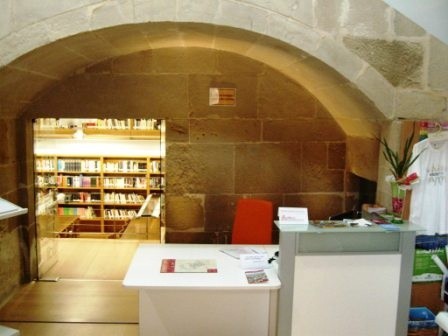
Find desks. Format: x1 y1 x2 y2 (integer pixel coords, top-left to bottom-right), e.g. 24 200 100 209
54 214 81 237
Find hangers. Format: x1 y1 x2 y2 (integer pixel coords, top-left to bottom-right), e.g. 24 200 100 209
430 121 448 144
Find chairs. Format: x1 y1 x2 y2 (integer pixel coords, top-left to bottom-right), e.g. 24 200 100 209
166 193 207 243
232 198 273 245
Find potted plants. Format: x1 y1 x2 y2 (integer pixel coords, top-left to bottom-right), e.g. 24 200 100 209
373 118 430 224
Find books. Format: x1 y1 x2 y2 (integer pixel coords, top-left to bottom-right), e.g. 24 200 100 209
150 177 161 188
34 159 54 171
36 174 101 188
103 176 146 188
103 159 147 172
150 160 160 173
103 192 145 203
104 209 137 219
58 159 101 172
57 191 101 203
58 208 96 220
159 258 218 273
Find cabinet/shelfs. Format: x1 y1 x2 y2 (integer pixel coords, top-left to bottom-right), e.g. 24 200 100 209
34 118 163 232
